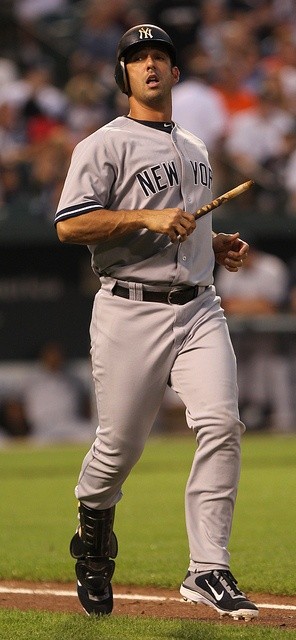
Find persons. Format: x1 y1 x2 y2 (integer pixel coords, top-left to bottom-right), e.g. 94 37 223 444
264 1 296 215
53 23 118 184
5 337 92 442
214 227 290 430
0 22 52 215
13 0 263 22
178 23 262 191
52 23 264 622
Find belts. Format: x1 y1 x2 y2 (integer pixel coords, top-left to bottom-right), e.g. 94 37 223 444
112 284 209 306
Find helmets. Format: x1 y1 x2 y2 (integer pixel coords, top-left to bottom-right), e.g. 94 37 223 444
113 24 175 96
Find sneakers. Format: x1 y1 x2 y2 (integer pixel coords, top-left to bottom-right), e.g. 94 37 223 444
180 569 259 622
75 559 114 617
